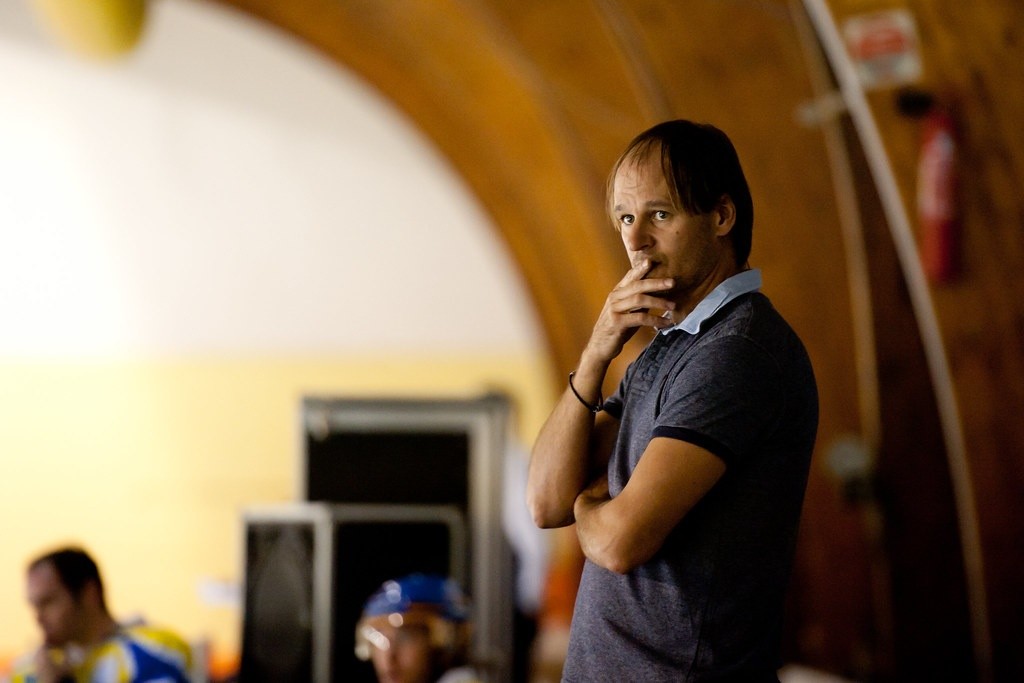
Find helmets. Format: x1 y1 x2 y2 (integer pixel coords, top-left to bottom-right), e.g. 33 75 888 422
354 574 475 658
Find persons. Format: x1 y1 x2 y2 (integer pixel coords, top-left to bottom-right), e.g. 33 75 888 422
12 547 213 683
355 575 487 683
525 120 819 683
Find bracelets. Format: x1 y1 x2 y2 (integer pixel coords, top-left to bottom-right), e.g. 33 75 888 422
570 371 605 414
